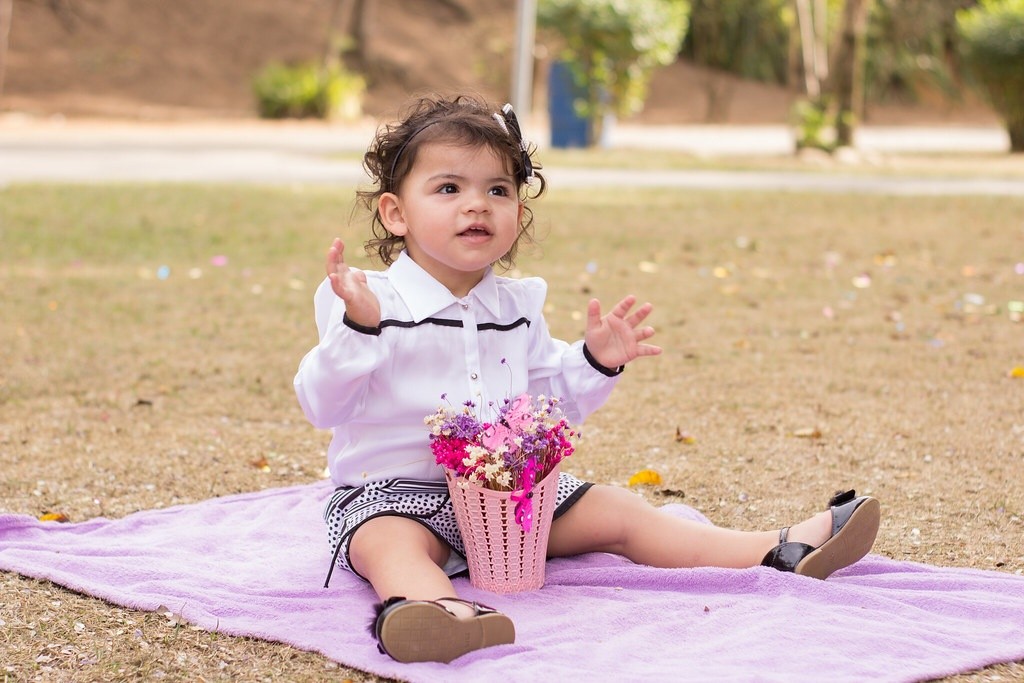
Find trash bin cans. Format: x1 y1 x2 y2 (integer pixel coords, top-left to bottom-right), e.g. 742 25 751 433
548 59 591 148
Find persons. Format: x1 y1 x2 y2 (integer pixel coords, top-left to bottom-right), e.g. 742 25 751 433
291 97 881 666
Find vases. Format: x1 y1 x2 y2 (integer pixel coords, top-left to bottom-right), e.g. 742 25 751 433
444 462 560 595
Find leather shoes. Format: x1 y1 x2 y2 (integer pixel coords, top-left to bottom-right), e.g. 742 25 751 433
364 596 515 663
761 488 880 580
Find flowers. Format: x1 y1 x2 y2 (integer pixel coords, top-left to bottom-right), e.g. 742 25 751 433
423 358 582 533
